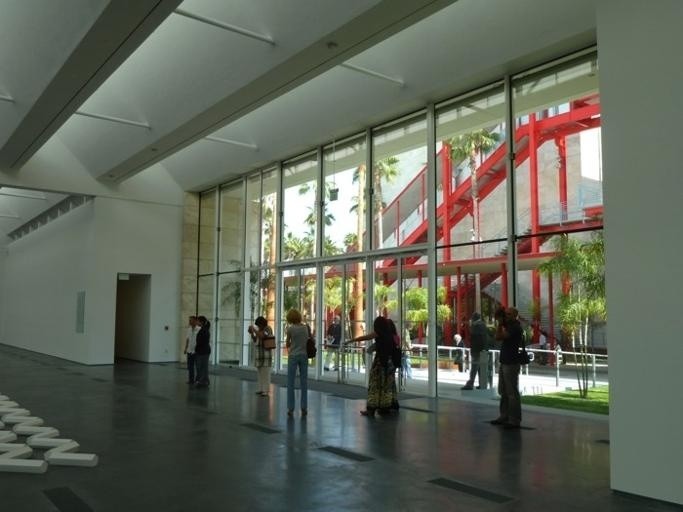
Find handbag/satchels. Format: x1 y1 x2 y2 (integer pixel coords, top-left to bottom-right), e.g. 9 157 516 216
519 350 530 365
263 335 276 349
393 347 402 368
307 339 317 358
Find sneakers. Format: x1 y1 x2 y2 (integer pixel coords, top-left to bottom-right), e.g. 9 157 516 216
360 409 398 416
505 423 521 428
287 410 293 418
302 408 307 416
187 380 208 386
460 386 472 389
491 420 504 424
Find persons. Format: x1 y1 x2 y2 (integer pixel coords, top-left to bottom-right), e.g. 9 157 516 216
184 316 201 384
453 312 534 390
342 316 413 419
553 338 563 366
194 316 211 386
539 330 547 365
286 309 310 415
248 316 273 397
491 307 524 430
324 315 342 371
